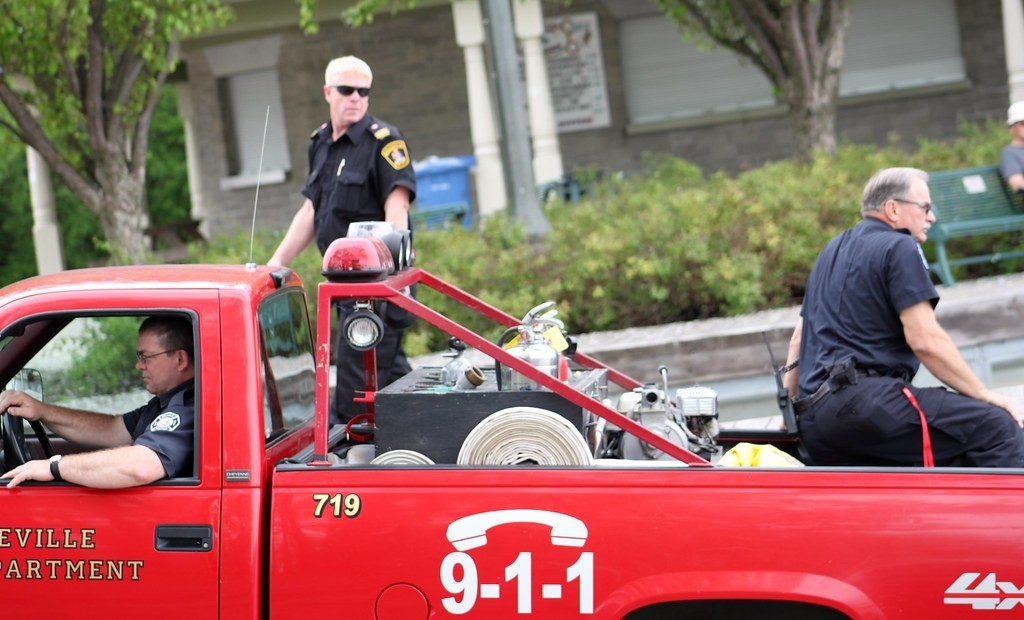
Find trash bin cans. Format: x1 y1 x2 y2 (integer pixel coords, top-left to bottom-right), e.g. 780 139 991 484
408 153 470 231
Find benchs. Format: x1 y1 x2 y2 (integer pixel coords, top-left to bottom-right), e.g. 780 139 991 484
918 164 1024 287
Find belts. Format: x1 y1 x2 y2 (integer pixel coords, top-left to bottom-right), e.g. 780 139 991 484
790 368 909 414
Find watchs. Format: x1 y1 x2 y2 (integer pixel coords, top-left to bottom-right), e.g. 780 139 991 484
49 454 61 477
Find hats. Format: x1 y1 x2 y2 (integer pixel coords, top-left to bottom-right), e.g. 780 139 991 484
1005 102 1024 125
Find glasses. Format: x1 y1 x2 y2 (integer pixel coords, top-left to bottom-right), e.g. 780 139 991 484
892 199 932 214
331 86 371 97
136 348 181 364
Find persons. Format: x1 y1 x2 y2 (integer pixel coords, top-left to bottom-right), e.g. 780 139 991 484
266 54 417 429
997 100 1024 193
0 312 195 489
781 167 1024 468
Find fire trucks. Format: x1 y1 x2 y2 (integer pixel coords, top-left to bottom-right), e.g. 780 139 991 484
0 222 1024 620
